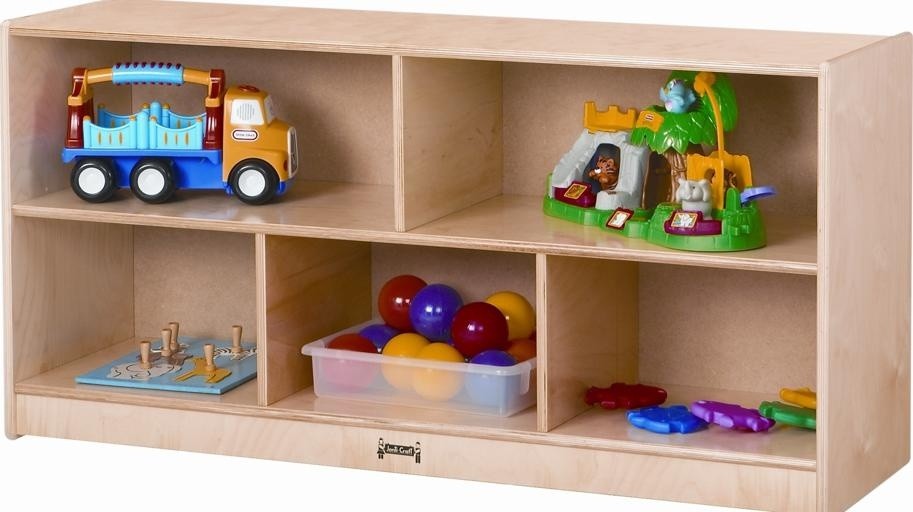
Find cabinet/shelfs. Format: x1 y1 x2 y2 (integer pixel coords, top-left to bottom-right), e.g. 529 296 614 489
0 0 913 512
